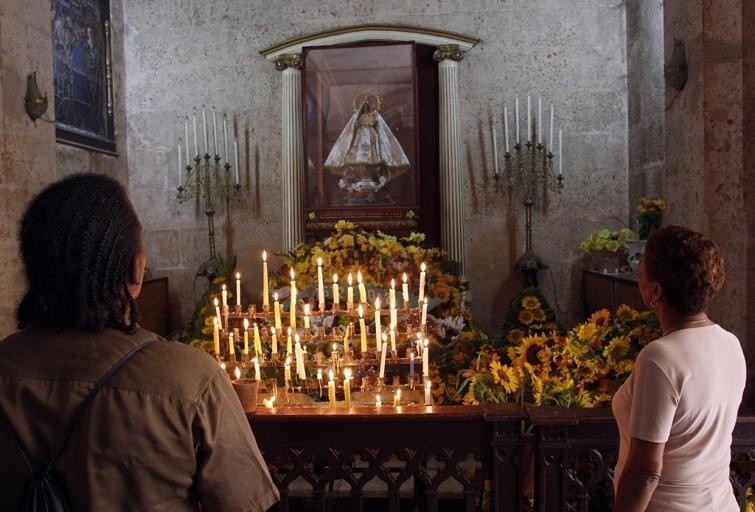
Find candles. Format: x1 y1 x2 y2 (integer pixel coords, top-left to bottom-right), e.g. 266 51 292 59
211 250 433 409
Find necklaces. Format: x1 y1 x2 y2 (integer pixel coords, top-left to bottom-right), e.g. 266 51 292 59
661 318 709 336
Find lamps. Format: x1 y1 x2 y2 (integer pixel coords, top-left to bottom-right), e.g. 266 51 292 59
175 104 241 261
492 92 564 288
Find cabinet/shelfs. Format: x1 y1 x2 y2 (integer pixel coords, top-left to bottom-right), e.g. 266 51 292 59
573 267 648 326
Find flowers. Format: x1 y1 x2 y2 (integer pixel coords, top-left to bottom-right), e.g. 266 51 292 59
636 198 665 239
580 228 637 257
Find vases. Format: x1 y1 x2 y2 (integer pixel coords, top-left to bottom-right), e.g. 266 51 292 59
590 246 631 273
625 240 648 273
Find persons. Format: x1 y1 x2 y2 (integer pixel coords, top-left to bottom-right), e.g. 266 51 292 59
0 175 280 511
612 225 747 512
